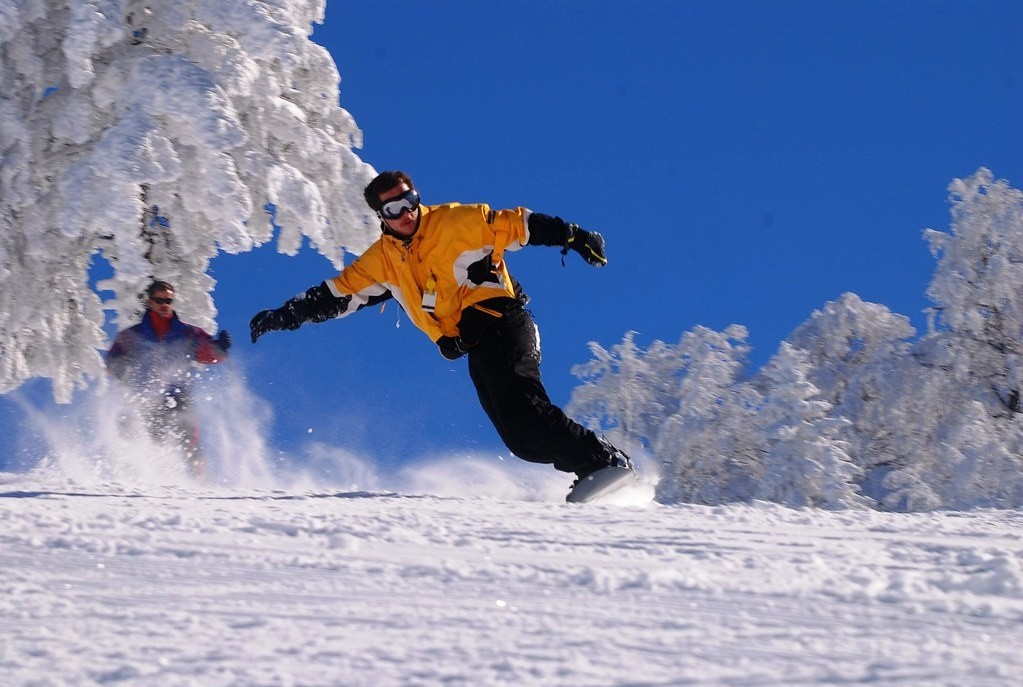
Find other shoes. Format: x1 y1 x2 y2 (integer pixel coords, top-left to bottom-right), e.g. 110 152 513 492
570 439 629 485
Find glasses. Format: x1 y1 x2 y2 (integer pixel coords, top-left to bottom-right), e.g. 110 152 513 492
149 297 173 304
380 189 420 220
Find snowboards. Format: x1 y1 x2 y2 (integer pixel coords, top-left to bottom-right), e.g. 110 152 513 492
566 466 635 503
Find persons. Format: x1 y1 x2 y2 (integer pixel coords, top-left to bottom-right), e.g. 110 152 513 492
250 170 631 489
103 281 232 475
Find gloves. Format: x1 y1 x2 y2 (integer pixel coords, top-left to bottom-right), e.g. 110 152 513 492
250 301 301 343
218 329 232 353
550 216 608 268
